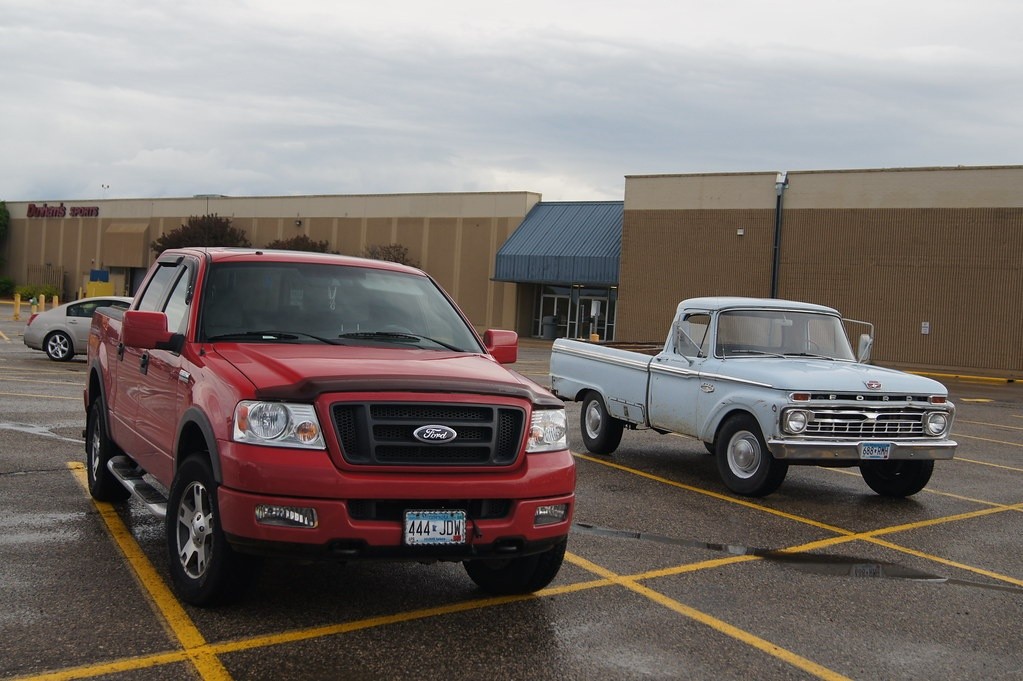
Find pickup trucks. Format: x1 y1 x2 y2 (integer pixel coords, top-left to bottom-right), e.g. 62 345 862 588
83 247 576 609
550 296 958 497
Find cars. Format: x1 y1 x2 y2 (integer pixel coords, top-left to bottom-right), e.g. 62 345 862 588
24 297 135 362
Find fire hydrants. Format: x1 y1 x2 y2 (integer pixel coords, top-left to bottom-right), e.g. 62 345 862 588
29 297 40 314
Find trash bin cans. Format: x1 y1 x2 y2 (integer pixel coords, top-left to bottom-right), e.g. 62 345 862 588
542 315 558 340
